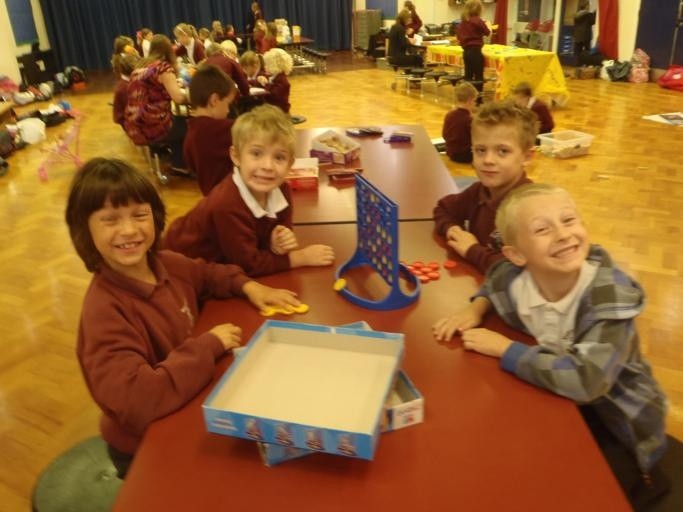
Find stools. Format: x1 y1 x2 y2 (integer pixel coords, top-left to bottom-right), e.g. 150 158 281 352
140 140 175 186
293 44 333 74
32 433 125 512
384 63 498 109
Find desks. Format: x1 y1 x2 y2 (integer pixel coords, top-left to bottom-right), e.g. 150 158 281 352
16 49 56 86
108 218 635 512
424 43 572 112
232 31 316 71
278 123 462 225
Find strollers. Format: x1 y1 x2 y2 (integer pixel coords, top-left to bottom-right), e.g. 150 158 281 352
3 109 89 183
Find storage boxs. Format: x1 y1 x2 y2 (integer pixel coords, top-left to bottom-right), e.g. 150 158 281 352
535 129 595 160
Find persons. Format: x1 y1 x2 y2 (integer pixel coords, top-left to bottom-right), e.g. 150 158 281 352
65 0 668 507
